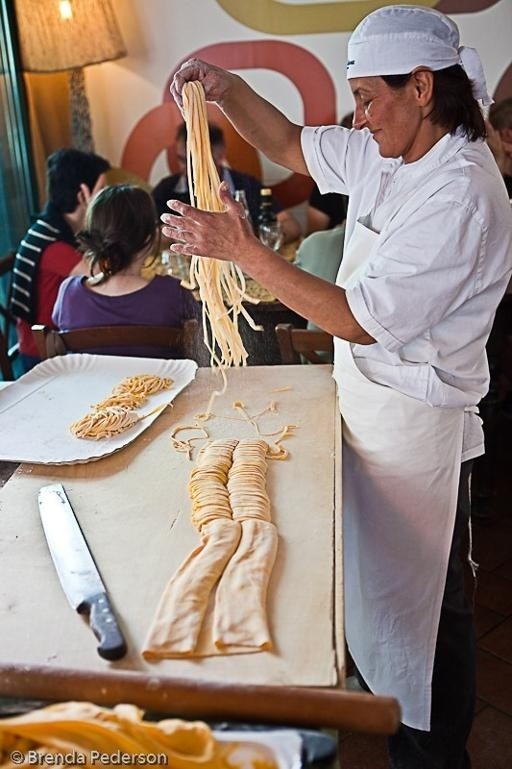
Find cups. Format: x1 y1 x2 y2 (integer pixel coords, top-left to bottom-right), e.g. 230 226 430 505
258 225 283 253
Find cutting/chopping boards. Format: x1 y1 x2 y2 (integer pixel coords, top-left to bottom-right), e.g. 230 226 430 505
0 364 345 691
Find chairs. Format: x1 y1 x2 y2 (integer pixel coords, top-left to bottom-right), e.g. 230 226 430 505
38 314 336 368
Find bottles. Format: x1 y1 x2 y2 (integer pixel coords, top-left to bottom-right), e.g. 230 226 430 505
233 189 252 226
258 189 277 249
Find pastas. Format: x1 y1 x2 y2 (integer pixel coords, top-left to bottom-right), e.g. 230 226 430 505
71 373 175 440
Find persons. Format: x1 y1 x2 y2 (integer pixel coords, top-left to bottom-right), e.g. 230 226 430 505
160 3 512 769
9 100 512 373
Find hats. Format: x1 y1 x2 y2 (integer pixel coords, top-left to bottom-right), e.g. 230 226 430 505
344 4 461 83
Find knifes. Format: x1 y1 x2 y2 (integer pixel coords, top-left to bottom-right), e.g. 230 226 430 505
36 481 128 660
